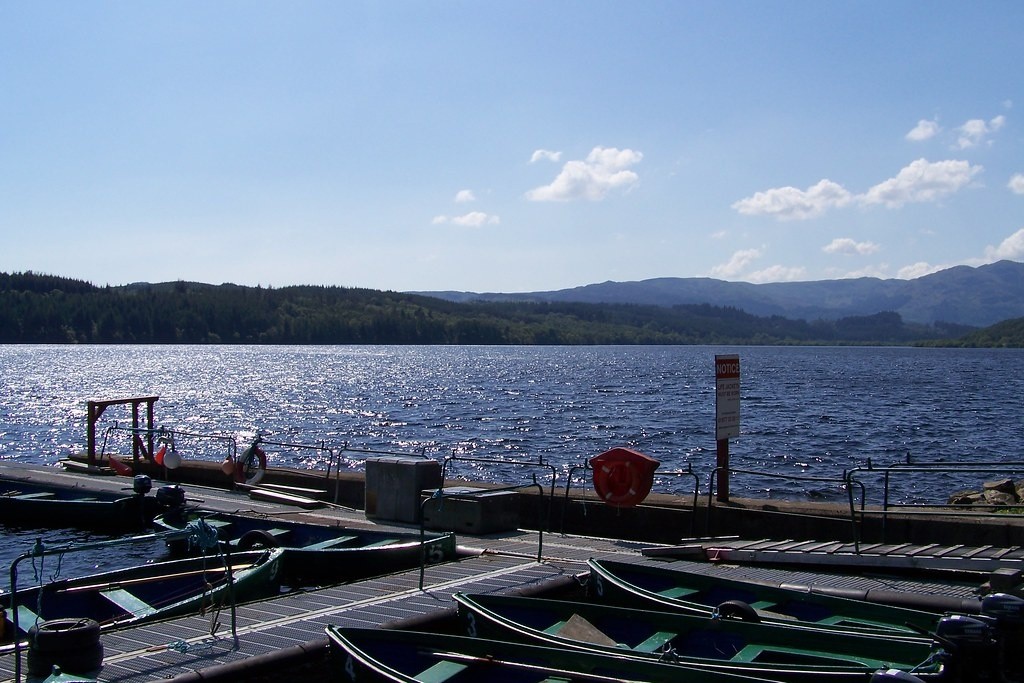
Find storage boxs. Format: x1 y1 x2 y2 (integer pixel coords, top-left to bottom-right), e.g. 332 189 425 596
365 456 442 522
418 486 522 534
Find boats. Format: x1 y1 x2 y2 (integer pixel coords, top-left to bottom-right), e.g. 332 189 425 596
0 477 456 647
325 557 1024 682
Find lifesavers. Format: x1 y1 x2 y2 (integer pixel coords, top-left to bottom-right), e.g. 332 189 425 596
236 530 282 582
27 643 104 674
236 448 266 491
26 618 101 650
708 600 760 659
600 461 639 502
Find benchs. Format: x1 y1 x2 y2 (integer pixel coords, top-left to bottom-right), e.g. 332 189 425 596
301 536 359 549
747 601 778 610
228 529 292 545
4 604 44 634
209 520 233 527
229 568 249 579
655 586 699 599
543 620 569 635
729 644 769 662
633 631 679 652
362 538 402 549
74 498 98 501
815 614 846 624
539 676 572 683
99 587 158 618
414 658 469 683
14 492 56 497
